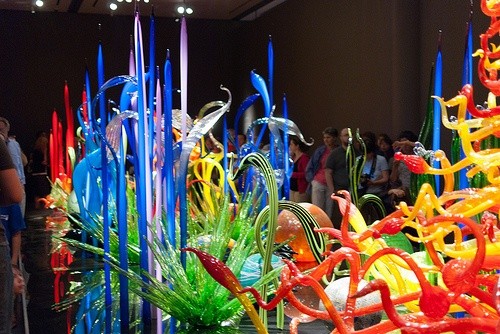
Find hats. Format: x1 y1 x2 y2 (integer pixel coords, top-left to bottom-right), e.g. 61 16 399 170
359 136 374 154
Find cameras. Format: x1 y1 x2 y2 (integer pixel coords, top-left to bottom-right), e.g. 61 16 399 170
359 172 368 186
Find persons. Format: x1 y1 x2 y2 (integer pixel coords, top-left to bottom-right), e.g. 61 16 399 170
229 127 418 227
0 117 27 334
